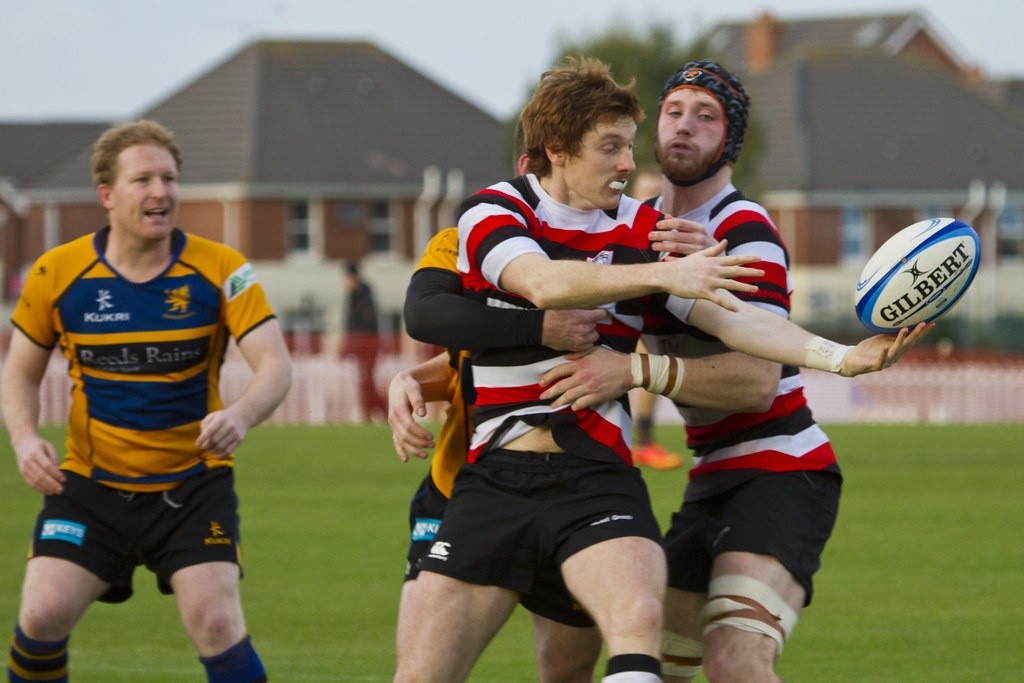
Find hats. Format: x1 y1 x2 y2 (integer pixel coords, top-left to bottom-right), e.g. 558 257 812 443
654 59 751 186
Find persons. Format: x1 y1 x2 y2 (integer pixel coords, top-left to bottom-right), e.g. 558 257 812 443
331 262 388 425
0 120 292 682
387 61 843 683
393 66 936 683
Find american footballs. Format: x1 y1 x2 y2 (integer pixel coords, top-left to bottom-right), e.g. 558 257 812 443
854 216 981 334
